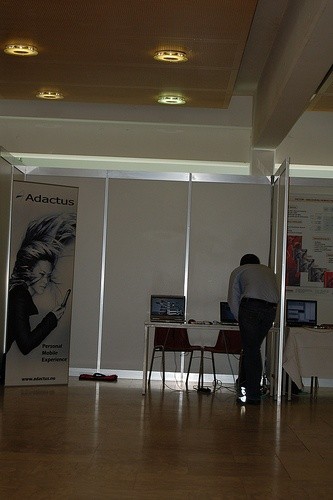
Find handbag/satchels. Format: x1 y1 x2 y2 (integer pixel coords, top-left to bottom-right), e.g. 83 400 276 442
78 373 118 382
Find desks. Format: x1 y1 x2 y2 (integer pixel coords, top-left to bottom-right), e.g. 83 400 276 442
142 321 280 401
283 327 333 401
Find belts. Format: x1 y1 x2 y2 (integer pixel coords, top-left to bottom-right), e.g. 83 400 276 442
242 298 277 306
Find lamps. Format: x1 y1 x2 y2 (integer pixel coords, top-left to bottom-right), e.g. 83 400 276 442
154 46 189 63
2 38 40 57
154 91 191 105
33 88 64 100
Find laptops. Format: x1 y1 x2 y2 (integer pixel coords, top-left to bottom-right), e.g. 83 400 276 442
286 299 319 328
220 302 239 326
150 295 185 325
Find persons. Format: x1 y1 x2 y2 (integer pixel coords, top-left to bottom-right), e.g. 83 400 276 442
0 214 76 355
227 254 279 402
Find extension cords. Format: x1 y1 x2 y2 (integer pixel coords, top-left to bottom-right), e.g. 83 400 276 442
198 388 211 392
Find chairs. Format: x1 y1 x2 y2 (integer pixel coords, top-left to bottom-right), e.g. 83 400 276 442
149 327 203 392
198 330 241 391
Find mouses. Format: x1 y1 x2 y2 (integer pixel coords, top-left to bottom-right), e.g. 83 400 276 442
188 319 196 324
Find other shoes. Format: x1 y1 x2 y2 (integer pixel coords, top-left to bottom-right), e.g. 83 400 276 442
236 397 261 406
236 379 248 389
